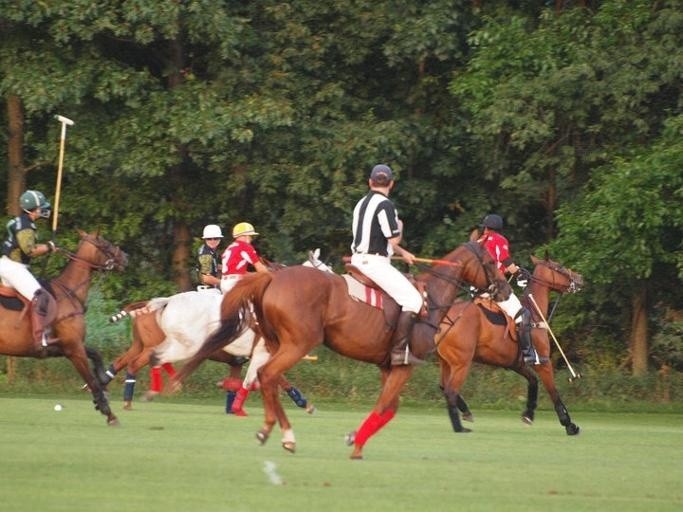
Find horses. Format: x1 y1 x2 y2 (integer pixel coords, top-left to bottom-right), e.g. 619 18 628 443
0 225 130 426
81 258 316 414
435 249 584 436
162 225 513 459
128 248 337 417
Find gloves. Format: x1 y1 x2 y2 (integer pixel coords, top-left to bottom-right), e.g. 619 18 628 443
48 240 57 252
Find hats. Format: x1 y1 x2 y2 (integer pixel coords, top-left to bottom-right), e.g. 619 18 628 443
370 164 393 181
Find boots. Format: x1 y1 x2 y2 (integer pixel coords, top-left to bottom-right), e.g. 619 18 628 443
515 321 548 365
391 311 427 365
31 300 60 352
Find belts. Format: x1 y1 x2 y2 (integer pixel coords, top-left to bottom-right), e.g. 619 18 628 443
201 287 214 289
223 276 241 279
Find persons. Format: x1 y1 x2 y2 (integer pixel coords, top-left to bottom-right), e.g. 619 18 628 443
466 213 548 370
221 218 277 334
195 224 225 296
346 160 427 371
2 185 66 349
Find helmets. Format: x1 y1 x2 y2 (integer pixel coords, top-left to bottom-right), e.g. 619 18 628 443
202 224 224 238
483 214 503 232
20 189 50 210
233 222 260 238
